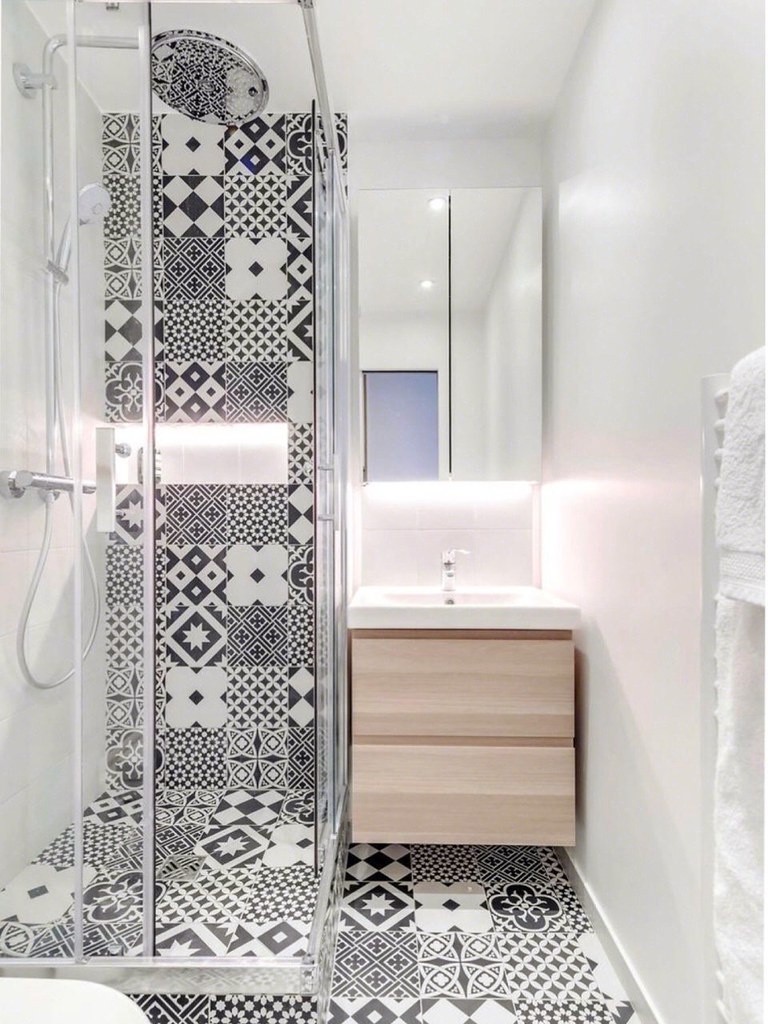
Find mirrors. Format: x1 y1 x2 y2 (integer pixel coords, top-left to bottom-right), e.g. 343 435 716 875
356 189 542 480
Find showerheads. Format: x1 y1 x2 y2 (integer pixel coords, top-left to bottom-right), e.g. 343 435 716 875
150 28 270 126
52 182 114 284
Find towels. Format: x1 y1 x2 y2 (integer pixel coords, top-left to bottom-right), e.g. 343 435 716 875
717 344 765 608
712 342 767 1024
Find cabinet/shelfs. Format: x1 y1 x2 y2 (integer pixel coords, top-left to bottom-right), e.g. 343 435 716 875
347 609 577 847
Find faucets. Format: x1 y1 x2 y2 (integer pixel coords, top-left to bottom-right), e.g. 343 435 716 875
14 468 96 501
436 546 469 599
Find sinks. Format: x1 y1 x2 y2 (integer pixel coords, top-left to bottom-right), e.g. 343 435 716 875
345 581 583 632
